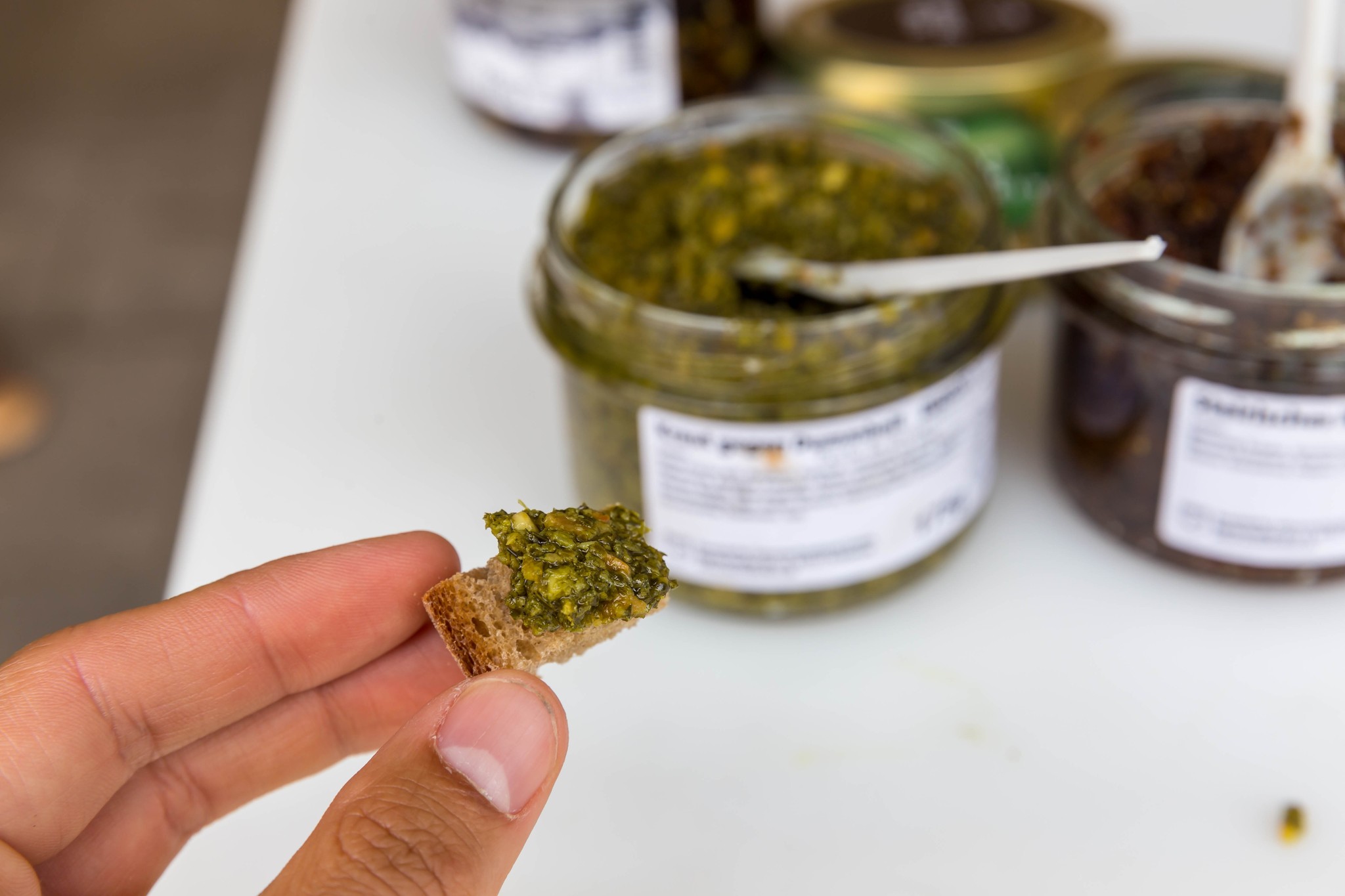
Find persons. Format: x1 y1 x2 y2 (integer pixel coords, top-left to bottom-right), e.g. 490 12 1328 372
2 533 570 896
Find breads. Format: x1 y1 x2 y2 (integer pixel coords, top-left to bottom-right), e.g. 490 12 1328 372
422 504 678 678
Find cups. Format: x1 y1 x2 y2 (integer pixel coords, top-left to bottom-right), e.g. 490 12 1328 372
437 0 677 142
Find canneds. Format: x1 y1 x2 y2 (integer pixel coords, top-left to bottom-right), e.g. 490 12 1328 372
788 0 1114 288
1044 59 1345 585
447 0 766 141
522 90 1012 616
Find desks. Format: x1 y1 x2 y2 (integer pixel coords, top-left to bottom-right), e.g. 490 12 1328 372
145 1 1345 896
